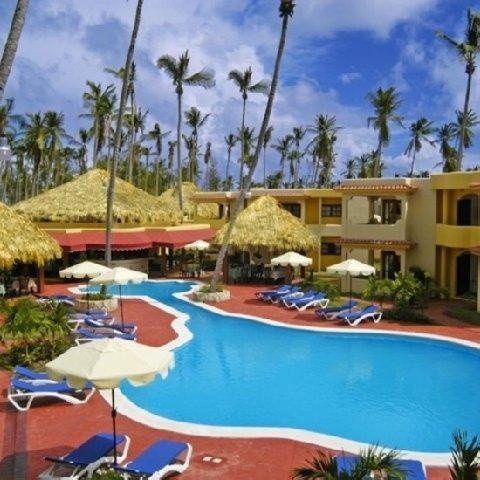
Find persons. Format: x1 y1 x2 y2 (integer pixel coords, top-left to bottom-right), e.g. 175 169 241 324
184 250 194 278
11 279 21 297
24 272 38 293
0 272 6 296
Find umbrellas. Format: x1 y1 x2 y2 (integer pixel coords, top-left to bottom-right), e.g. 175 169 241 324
58 261 111 311
271 251 313 293
45 336 176 467
325 258 377 313
89 266 150 334
185 240 211 252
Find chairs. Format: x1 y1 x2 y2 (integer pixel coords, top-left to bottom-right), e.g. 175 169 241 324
331 455 427 480
37 430 193 480
254 286 382 327
3 277 138 411
